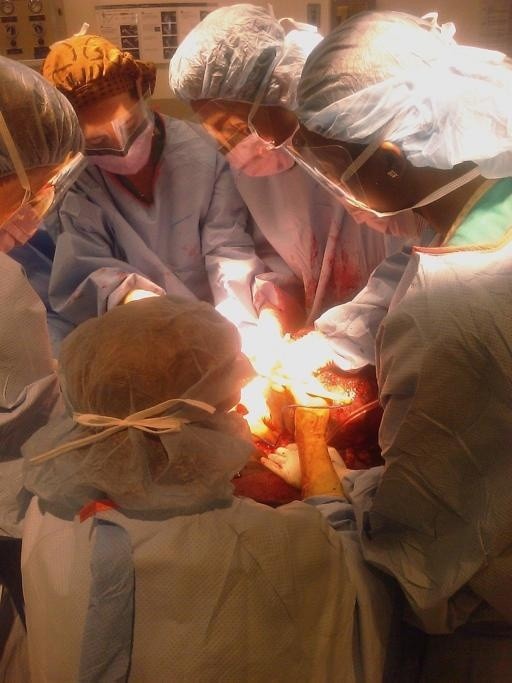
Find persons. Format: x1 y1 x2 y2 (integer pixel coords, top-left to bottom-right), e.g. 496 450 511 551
253 8 511 643
167 3 442 383
1 54 92 541
5 19 272 359
0 293 396 682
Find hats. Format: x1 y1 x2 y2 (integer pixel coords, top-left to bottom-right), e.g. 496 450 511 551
42 34 156 115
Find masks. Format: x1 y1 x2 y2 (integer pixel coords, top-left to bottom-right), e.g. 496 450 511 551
336 144 428 240
225 101 300 178
85 77 155 176
0 170 44 255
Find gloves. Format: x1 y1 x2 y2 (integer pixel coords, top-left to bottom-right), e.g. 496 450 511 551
259 442 349 491
